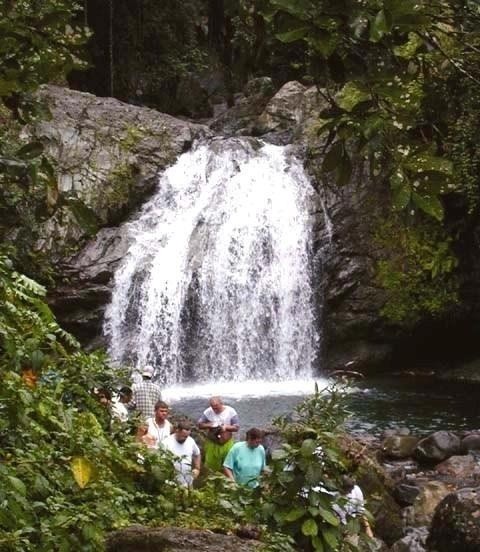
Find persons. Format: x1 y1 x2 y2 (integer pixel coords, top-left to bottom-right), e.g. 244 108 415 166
197 397 240 444
161 422 201 488
223 427 266 487
132 365 162 419
94 369 133 435
283 437 373 545
137 401 172 451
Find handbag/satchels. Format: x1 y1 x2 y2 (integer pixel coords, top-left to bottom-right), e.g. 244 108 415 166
216 429 233 445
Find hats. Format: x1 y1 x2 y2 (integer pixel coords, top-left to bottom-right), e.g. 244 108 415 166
142 365 154 378
